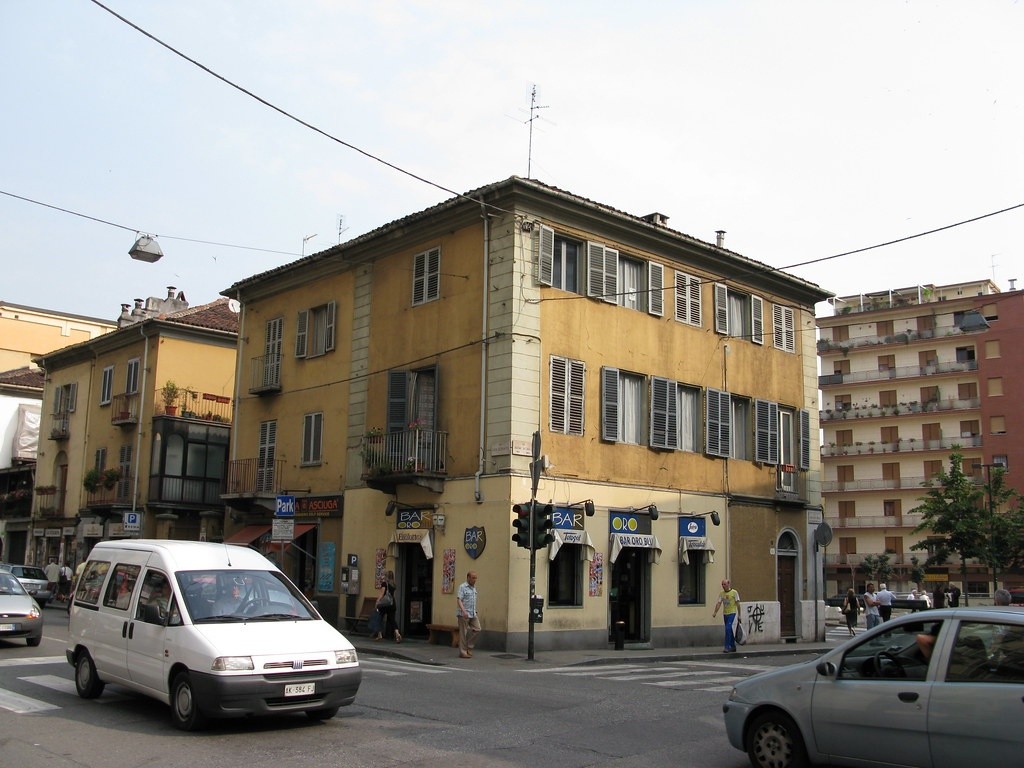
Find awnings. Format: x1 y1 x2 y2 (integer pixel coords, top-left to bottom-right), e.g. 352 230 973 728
269 524 316 559
221 524 272 556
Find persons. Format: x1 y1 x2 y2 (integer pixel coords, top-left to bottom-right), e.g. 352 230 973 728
44 558 63 602
990 589 1012 650
946 583 960 608
877 583 897 622
933 584 945 609
919 590 932 610
373 571 402 643
712 579 742 653
59 562 73 600
916 623 944 660
907 590 917 613
456 572 481 658
863 583 881 631
209 580 250 616
75 555 91 580
843 588 860 637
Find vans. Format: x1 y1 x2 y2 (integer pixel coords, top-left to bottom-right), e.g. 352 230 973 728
0 568 44 647
65 538 362 732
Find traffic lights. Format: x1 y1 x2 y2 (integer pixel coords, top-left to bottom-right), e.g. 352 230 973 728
512 502 530 547
533 503 554 549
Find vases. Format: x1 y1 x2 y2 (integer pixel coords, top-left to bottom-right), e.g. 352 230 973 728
414 430 424 438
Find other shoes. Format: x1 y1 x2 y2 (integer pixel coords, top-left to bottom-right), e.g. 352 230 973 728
376 636 383 640
467 650 472 656
460 652 471 658
396 634 402 643
49 596 54 603
852 629 856 636
723 649 729 653
61 596 66 603
728 648 736 652
849 634 852 637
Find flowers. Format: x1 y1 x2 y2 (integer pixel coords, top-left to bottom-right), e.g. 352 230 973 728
408 419 427 432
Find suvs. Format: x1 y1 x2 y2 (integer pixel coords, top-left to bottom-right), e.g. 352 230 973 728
0 561 53 609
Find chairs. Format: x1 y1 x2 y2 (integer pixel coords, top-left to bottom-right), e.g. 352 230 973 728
961 636 995 682
996 631 1024 683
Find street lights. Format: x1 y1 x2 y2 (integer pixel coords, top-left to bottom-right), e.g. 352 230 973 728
971 461 1006 606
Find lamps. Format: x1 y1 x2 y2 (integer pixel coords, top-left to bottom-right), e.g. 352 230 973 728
959 310 991 332
128 235 164 263
567 499 595 516
634 502 659 520
696 511 720 526
385 500 416 516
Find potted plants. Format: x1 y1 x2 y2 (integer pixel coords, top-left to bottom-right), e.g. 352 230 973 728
34 485 57 495
405 457 424 471
180 385 232 423
120 388 130 420
162 380 179 416
358 426 392 472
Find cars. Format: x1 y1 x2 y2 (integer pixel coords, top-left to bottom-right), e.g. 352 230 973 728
723 606 1024 768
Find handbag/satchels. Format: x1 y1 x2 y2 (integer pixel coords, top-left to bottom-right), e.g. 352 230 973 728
842 603 851 615
376 593 393 611
59 575 67 585
734 621 746 645
